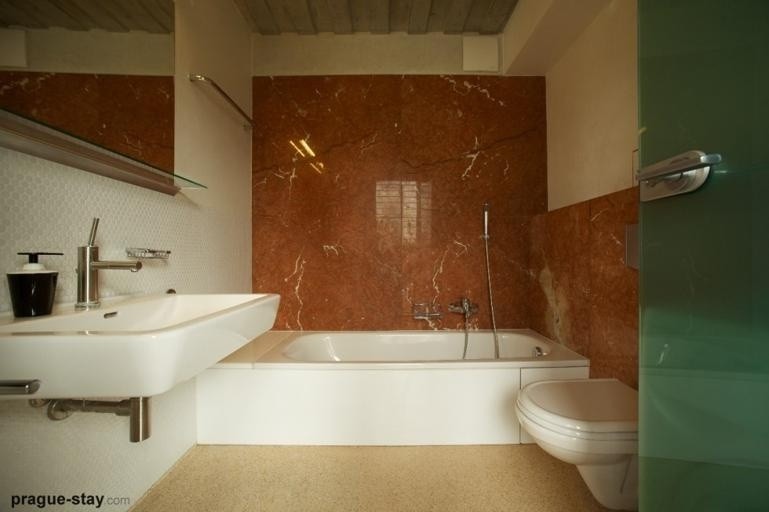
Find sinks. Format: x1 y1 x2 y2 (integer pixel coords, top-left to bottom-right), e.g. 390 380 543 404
0 291 280 401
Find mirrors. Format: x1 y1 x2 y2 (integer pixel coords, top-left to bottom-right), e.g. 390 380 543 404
1 0 207 197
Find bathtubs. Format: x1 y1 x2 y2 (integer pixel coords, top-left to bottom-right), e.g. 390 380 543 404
196 329 591 446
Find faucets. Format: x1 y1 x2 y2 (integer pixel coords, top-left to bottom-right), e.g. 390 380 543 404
76 218 141 308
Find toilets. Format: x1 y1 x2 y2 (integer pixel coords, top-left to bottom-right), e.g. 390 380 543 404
516 377 638 511
